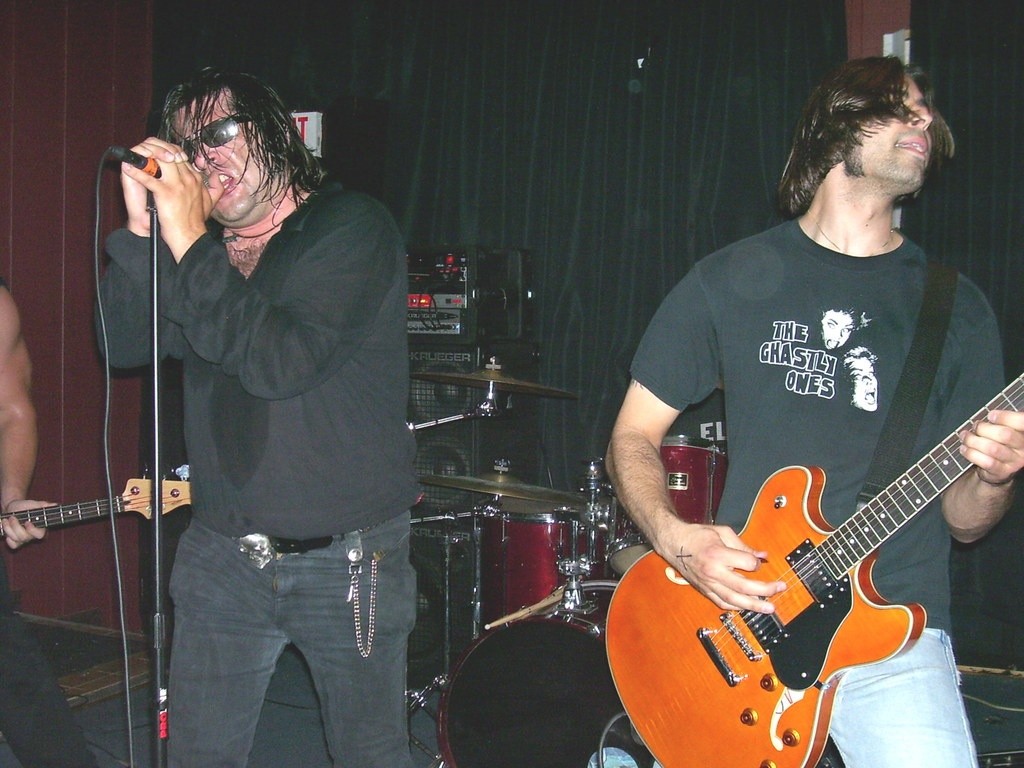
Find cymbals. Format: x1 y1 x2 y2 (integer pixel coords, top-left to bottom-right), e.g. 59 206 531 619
412 360 567 398
415 464 585 507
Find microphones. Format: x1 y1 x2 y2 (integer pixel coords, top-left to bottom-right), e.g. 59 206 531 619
108 146 210 188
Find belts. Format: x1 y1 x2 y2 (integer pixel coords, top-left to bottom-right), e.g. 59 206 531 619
238 525 372 570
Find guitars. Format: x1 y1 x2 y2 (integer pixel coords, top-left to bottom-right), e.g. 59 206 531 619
605 370 1024 766
0 465 190 536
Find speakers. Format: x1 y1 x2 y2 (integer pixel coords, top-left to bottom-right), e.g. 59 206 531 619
407 339 542 673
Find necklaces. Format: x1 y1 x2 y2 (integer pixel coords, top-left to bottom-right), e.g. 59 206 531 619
221 222 286 243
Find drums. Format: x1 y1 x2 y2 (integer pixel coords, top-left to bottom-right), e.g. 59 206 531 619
609 435 727 574
955 662 1024 768
503 510 608 637
437 603 624 767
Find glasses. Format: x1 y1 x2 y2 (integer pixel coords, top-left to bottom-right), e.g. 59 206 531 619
178 115 252 164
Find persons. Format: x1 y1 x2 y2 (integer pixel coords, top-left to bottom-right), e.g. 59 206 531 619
601 55 1024 768
91 61 425 768
0 281 102 768
